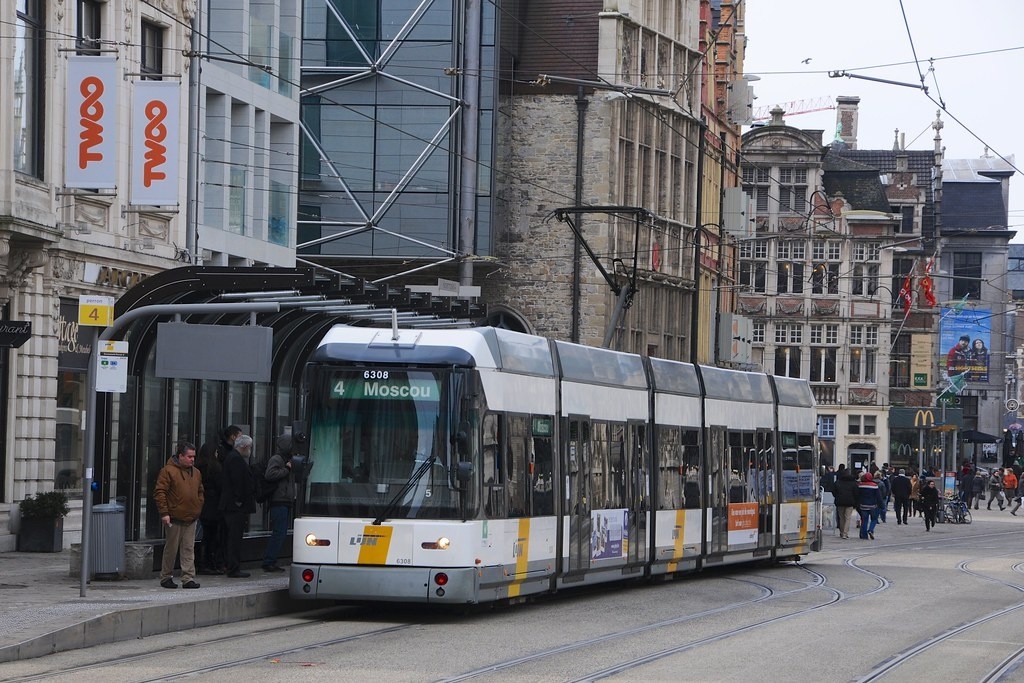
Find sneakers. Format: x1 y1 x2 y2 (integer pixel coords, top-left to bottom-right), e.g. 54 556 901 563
183 581 200 588
161 579 178 588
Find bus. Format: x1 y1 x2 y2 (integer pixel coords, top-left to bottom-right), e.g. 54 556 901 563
288 206 823 607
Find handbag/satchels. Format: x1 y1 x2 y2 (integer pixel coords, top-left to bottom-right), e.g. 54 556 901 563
999 490 1006 501
980 494 985 500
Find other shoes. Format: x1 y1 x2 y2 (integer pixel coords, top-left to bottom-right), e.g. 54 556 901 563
228 571 250 578
869 531 875 540
974 506 979 510
840 532 849 539
1011 510 1016 516
266 567 284 573
198 567 224 575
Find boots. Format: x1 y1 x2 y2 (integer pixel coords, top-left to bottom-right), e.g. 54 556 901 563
987 503 991 510
998 503 1005 510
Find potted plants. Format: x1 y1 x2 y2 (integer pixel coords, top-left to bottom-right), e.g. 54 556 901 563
17 491 71 553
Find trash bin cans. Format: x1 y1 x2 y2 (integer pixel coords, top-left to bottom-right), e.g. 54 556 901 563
90 504 125 582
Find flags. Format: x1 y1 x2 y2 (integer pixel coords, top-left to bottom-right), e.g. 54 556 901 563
920 260 935 308
899 273 914 318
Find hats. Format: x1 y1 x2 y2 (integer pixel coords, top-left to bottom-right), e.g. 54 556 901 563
959 334 970 343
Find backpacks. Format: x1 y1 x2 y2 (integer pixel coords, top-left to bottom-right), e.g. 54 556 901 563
250 451 287 504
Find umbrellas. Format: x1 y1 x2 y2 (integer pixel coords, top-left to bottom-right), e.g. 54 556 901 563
955 429 1002 463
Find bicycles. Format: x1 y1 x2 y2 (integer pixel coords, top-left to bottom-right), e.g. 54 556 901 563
946 491 972 524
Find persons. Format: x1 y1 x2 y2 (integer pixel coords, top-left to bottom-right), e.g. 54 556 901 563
154 441 204 589
200 424 293 578
819 448 1024 541
946 336 989 380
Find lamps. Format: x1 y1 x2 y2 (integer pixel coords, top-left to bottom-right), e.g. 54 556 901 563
185 249 212 261
63 222 91 235
128 236 155 249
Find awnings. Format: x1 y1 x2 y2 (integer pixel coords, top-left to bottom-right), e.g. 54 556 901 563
887 405 964 429
